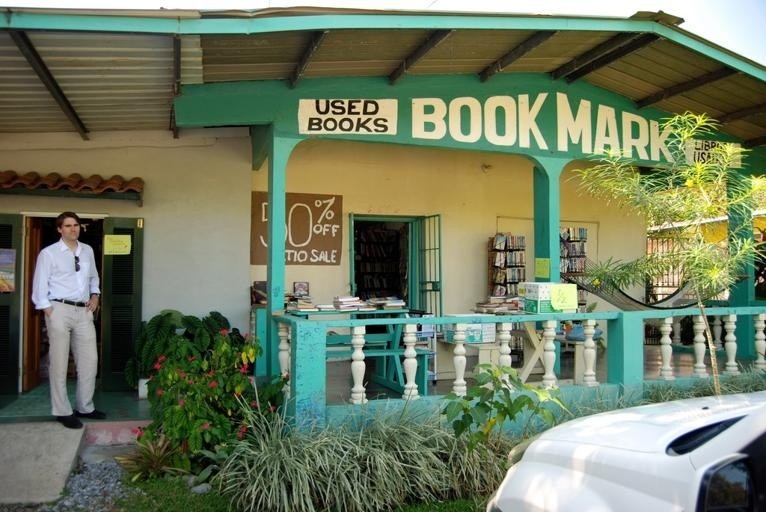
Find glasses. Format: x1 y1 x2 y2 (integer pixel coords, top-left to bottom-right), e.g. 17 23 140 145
75 256 80 271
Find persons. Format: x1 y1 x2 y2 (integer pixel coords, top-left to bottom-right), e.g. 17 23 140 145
31 210 107 429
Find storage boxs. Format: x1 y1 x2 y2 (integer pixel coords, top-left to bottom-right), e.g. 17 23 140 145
443 313 496 344
518 282 578 315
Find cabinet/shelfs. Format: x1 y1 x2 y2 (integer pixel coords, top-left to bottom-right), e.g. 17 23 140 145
560 238 587 353
487 236 527 367
354 221 408 319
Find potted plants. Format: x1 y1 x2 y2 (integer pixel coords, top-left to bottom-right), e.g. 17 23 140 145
124 310 232 399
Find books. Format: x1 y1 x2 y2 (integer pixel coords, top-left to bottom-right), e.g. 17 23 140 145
251 226 406 317
487 232 526 351
560 227 588 312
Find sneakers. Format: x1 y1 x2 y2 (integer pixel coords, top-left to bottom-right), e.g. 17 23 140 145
56 415 82 428
75 409 106 419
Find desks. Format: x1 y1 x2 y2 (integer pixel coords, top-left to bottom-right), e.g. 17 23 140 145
498 310 547 385
289 309 409 394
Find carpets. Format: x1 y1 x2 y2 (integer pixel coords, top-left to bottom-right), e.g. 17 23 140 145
0 380 100 418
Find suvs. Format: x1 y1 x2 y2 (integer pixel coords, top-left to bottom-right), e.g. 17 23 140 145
484 390 766 512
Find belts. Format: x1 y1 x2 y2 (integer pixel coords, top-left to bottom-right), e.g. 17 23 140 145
54 299 85 307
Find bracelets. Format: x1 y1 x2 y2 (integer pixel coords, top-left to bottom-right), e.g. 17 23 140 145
90 293 100 299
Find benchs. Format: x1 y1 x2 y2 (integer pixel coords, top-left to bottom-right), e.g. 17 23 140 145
437 339 501 374
496 329 597 385
326 349 429 396
326 334 392 385
